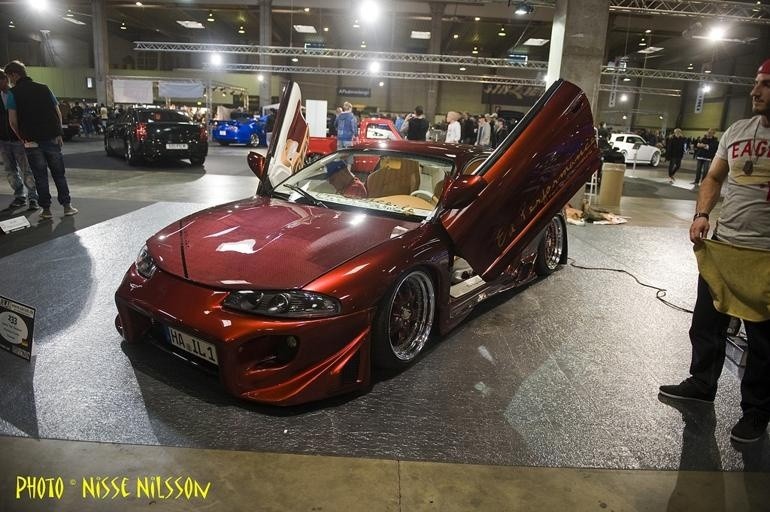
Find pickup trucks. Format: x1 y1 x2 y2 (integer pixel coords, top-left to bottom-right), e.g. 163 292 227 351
304 118 404 174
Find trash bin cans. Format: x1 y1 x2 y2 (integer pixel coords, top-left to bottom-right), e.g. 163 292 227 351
600 163 625 213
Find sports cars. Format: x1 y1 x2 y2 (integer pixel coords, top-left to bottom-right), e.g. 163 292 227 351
112 76 604 407
208 116 268 147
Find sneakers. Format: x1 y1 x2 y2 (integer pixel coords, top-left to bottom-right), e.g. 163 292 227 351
8 198 27 208
63 205 78 217
28 200 39 211
39 209 53 219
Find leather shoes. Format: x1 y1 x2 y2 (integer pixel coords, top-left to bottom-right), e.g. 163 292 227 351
660 374 719 404
731 415 765 444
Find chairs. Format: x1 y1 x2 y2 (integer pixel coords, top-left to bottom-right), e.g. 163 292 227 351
385 159 419 195
463 160 486 177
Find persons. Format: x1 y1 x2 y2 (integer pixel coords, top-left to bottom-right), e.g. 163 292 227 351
324 101 720 199
0 59 78 219
660 62 769 444
62 100 276 148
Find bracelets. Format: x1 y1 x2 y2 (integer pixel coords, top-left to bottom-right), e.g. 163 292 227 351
694 212 711 222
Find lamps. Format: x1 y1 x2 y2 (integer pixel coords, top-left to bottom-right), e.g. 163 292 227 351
515 4 532 16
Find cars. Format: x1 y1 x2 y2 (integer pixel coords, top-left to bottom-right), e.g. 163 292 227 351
611 132 661 167
104 104 209 167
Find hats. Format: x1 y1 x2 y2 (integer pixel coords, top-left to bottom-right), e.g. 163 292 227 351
758 59 770 75
